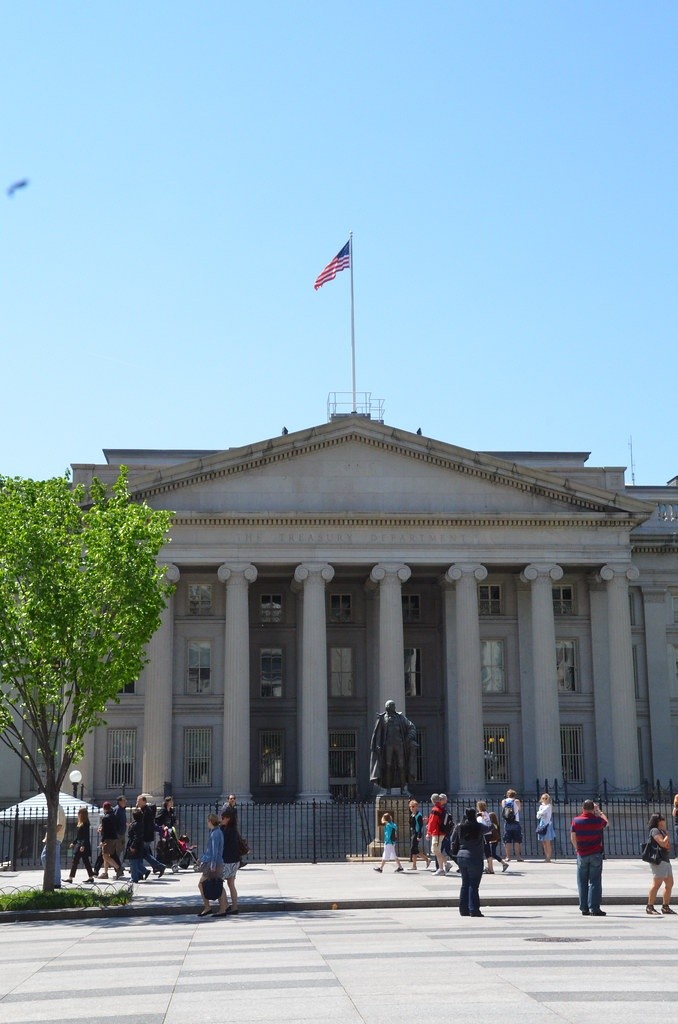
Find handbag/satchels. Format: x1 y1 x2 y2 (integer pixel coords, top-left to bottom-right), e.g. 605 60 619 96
239 836 251 856
535 824 547 836
201 870 224 900
640 828 663 865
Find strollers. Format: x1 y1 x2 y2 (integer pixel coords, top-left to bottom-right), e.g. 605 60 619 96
160 826 202 874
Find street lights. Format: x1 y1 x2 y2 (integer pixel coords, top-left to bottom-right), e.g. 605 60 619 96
69 770 83 797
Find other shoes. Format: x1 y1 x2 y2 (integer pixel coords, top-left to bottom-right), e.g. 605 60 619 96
97 873 108 879
461 912 475 917
145 869 151 880
137 875 144 880
63 879 72 883
582 910 590 915
470 911 484 917
661 906 677 914
198 909 212 916
646 904 661 915
116 866 123 880
82 878 94 883
591 911 606 916
127 878 139 883
159 864 166 878
373 858 551 876
226 909 238 915
211 912 226 917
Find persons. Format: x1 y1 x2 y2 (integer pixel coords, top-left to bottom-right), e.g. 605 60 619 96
222 795 236 813
537 793 557 863
645 813 677 915
124 795 175 883
178 835 198 864
370 700 416 796
41 804 67 888
196 808 241 917
407 800 431 870
570 799 609 916
451 807 489 917
62 808 94 884
426 793 460 875
373 813 404 873
476 801 509 874
501 789 525 862
93 795 127 880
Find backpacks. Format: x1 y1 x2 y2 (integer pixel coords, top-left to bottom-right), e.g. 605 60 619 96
438 806 454 834
502 799 514 823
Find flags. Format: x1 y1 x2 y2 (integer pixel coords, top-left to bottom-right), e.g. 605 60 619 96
314 240 349 291
